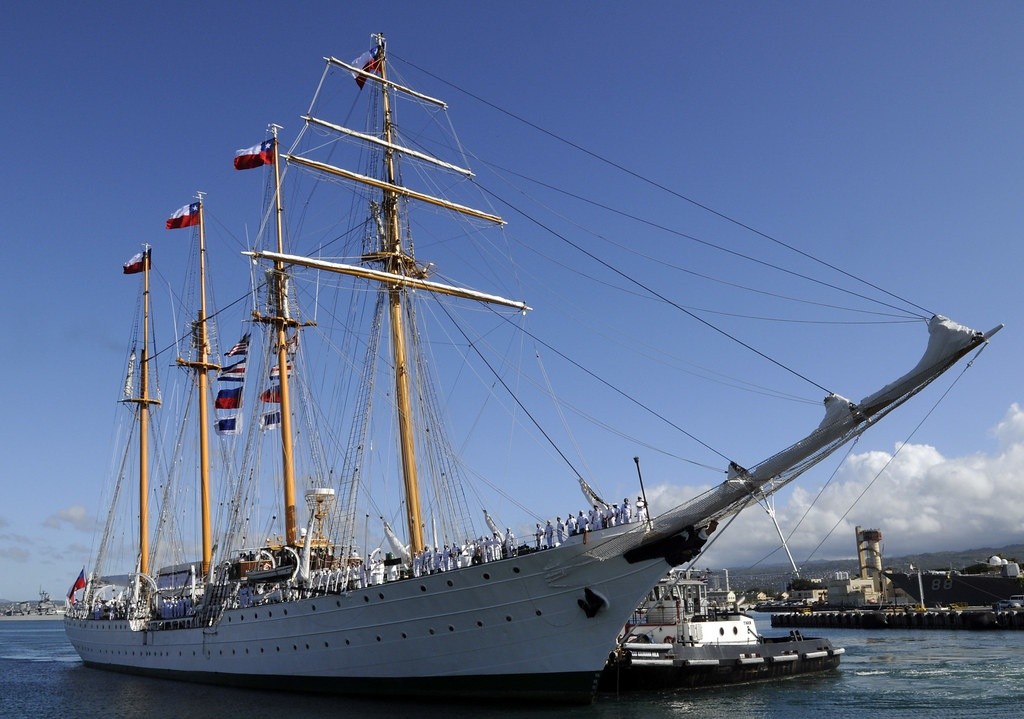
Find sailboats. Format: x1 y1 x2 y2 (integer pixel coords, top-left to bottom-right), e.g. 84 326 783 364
60 28 1006 704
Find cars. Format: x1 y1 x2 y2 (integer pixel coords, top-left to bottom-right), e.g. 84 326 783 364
997 599 1021 608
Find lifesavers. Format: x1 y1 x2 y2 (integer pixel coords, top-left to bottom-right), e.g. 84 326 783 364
663 636 674 644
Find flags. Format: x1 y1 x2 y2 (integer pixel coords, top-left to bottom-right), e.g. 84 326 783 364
259 408 281 432
213 414 243 435
224 333 250 357
165 202 200 230
214 386 243 409
270 357 294 380
234 139 275 170
350 45 382 91
272 338 296 354
66 569 87 604
260 384 280 404
123 248 151 274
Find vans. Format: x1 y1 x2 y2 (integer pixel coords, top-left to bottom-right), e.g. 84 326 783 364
1010 595 1024 608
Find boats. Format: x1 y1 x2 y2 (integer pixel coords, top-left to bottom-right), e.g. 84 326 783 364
880 554 1024 608
596 560 846 691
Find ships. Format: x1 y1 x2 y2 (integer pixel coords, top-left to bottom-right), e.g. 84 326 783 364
0 585 66 620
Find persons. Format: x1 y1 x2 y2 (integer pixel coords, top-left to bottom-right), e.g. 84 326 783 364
239 554 374 609
535 496 646 548
73 599 80 609
95 599 114 620
160 595 192 618
217 358 246 382
412 527 514 577
